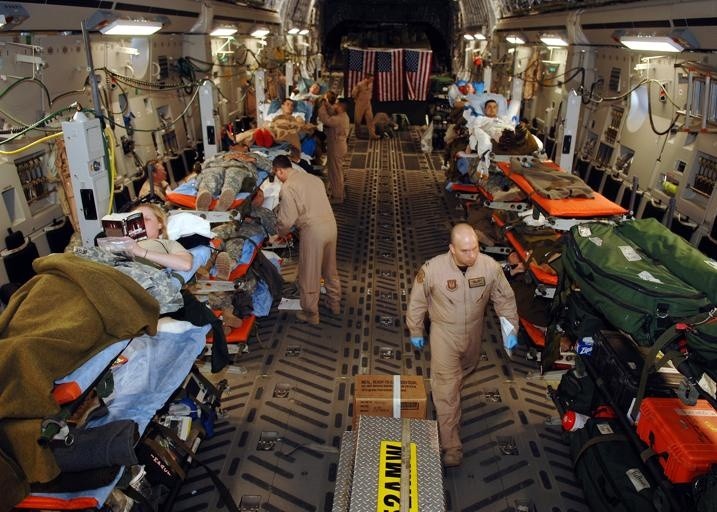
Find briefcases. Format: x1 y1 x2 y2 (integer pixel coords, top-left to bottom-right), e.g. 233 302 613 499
591 329 693 411
637 395 717 485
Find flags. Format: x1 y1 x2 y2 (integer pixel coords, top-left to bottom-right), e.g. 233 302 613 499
347 47 374 99
372 48 404 101
403 50 432 102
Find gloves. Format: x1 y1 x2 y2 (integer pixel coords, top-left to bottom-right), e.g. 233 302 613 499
504 337 519 352
411 336 427 352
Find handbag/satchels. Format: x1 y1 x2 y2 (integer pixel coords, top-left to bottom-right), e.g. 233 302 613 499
555 368 593 416
561 213 716 364
570 419 663 512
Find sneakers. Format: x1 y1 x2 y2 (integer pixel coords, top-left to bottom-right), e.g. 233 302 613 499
221 304 243 334
195 188 214 212
294 308 322 328
356 131 381 140
215 188 236 212
442 448 464 469
215 250 234 282
329 196 345 205
322 296 342 316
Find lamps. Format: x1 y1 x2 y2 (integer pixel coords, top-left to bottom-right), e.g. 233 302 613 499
247 26 271 38
614 28 697 56
210 24 239 38
539 33 570 48
505 33 528 45
87 9 171 42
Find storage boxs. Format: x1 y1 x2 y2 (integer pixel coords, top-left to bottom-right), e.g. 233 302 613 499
351 374 428 428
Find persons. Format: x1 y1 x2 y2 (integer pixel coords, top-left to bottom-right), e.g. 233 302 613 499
351 73 381 140
406 224 519 468
453 83 477 110
194 84 350 326
97 202 193 275
138 160 172 204
483 100 525 130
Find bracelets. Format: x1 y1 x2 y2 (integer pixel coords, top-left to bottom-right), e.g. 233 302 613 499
143 246 151 259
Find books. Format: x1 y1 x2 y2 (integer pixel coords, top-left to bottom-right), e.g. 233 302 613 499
101 212 149 243
